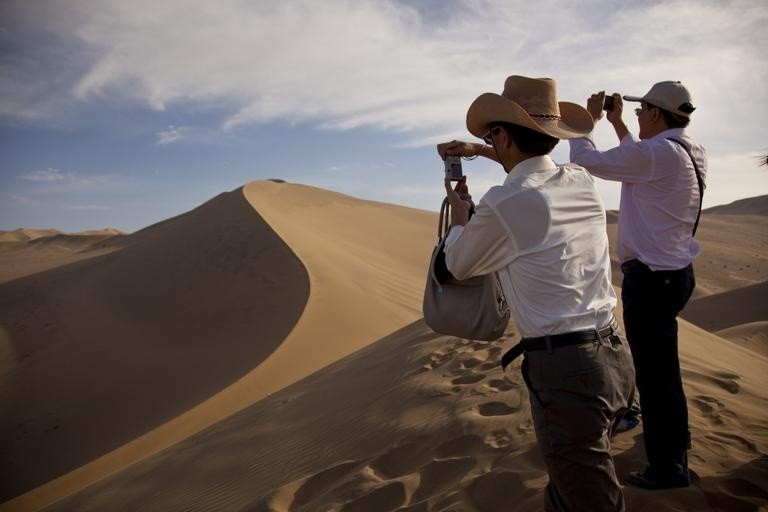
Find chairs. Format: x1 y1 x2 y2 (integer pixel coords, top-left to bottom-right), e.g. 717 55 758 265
626 470 689 490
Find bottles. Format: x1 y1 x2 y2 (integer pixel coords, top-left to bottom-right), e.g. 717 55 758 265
623 81 692 118
466 75 594 139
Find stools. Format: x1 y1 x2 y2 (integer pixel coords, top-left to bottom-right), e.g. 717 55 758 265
501 314 618 371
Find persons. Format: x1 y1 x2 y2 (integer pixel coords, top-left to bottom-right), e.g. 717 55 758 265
436 76 635 511
568 80 708 488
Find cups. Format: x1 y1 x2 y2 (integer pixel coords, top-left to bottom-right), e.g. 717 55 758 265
481 126 502 145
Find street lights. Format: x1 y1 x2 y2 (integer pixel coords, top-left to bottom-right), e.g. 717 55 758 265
423 195 511 342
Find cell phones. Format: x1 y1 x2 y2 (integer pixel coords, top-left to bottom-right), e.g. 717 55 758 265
603 95 615 111
445 156 462 181
